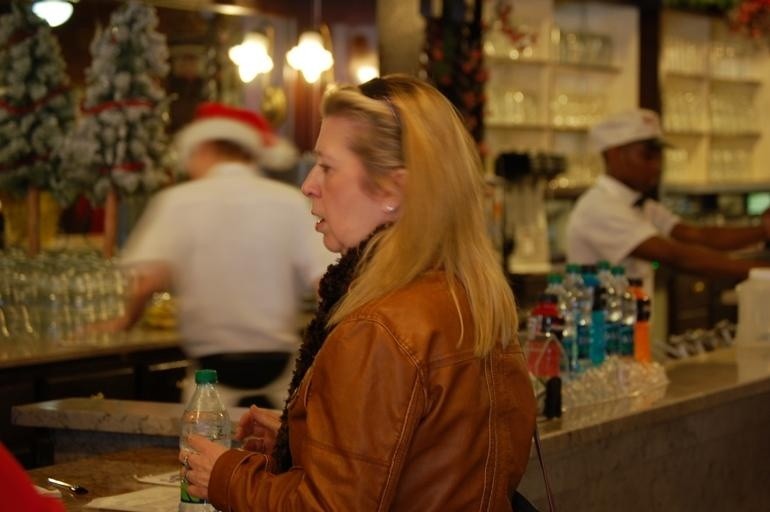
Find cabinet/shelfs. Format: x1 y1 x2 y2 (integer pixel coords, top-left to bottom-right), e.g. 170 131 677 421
174 366 231 512
733 266 770 347
518 262 652 385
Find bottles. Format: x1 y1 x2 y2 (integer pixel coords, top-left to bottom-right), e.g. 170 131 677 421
590 107 680 155
168 101 298 175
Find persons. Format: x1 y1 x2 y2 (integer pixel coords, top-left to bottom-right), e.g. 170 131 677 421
98 103 333 410
177 74 534 512
563 110 770 351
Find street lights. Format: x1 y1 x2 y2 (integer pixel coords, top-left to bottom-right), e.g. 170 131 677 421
194 350 291 390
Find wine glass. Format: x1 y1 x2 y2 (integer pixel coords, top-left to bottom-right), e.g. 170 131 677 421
183 455 191 467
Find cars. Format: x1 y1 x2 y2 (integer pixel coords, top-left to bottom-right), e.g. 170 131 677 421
0 234 182 354
